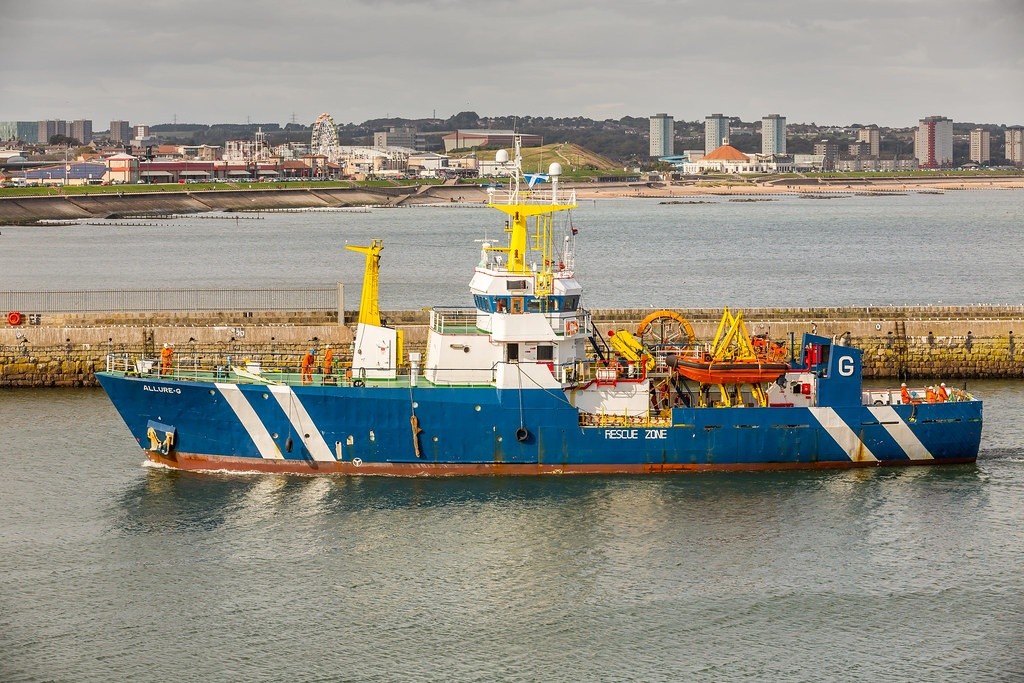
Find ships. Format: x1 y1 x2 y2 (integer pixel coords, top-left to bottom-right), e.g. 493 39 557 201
92 136 984 478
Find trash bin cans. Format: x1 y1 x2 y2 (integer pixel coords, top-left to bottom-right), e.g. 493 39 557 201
35 314 42 325
29 314 34 325
243 312 253 317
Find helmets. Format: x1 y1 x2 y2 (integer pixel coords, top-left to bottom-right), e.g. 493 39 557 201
941 383 946 386
928 386 934 389
310 350 314 355
164 343 168 349
326 345 330 349
901 383 908 387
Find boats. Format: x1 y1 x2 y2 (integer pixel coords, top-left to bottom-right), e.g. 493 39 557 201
663 356 790 385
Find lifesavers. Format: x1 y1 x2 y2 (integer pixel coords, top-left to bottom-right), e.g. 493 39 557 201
516 428 528 441
566 321 579 336
612 363 624 373
875 324 881 331
706 356 711 362
9 314 19 324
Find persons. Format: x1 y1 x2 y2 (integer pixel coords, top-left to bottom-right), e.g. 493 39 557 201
161 343 173 379
926 383 949 403
901 383 913 404
302 350 314 384
322 345 333 379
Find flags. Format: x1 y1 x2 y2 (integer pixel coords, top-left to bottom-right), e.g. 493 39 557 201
522 172 549 188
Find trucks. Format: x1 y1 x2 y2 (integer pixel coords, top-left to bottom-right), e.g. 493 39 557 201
11 177 27 188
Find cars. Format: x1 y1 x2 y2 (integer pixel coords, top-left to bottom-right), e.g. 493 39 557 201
0 180 15 188
178 176 330 184
